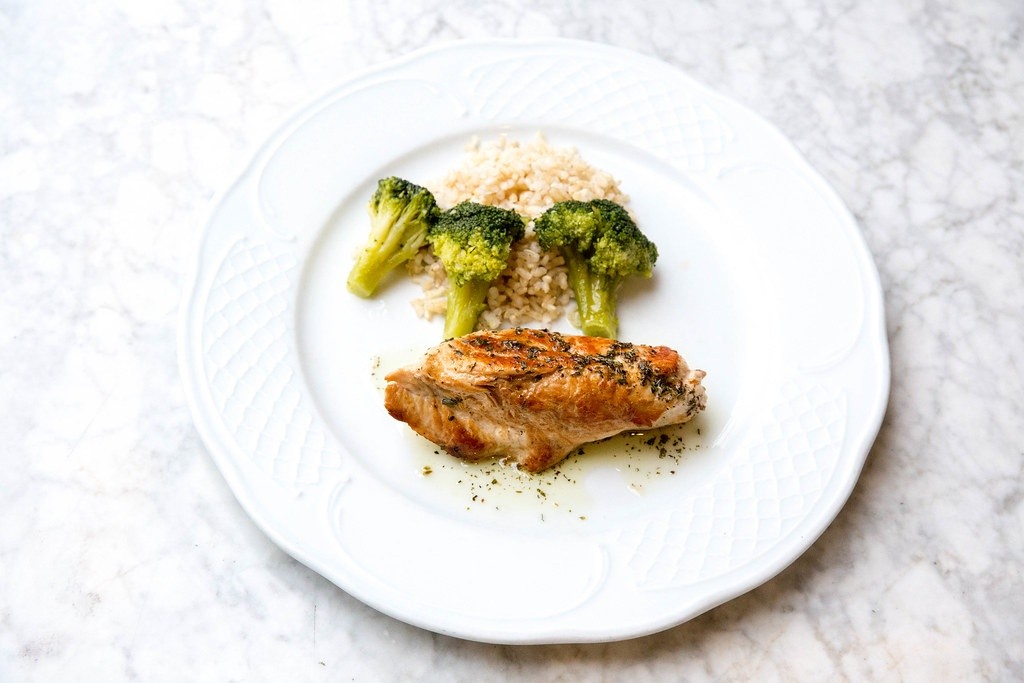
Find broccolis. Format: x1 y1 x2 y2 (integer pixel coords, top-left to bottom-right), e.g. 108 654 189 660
347 177 658 343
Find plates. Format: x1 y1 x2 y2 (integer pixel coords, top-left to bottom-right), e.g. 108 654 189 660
186 32 893 647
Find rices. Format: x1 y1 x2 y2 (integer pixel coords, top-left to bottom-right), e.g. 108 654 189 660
398 130 631 331
